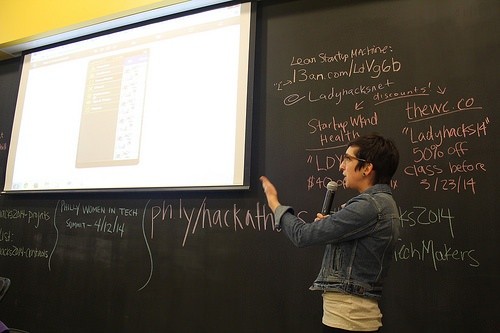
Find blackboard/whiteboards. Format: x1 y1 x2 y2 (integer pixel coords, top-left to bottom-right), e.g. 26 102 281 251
0 0 500 333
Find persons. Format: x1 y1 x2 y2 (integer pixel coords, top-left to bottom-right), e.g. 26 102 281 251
259 133 400 333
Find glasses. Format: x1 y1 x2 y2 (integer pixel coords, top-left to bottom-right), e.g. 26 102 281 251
344 153 369 164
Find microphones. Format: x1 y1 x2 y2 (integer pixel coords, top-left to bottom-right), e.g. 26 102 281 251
322 181 338 216
